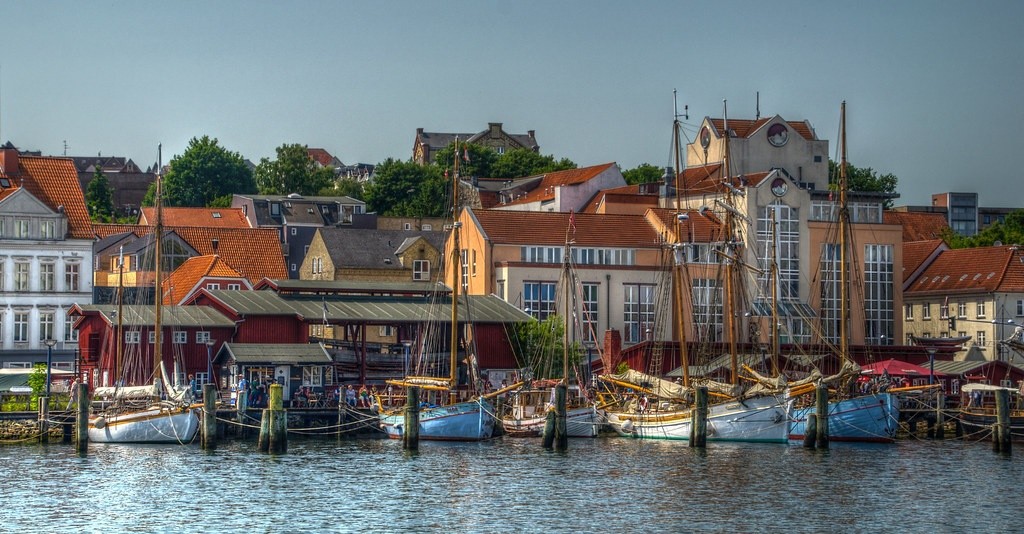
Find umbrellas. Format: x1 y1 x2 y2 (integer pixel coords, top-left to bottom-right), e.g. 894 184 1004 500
860 358 947 376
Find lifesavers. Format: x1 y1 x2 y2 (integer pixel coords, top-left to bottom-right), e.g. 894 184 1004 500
638 397 648 414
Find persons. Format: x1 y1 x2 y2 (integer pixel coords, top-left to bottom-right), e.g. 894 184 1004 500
112 376 125 387
501 380 508 397
66 376 80 411
188 374 198 403
295 384 310 407
335 384 378 407
639 394 648 415
963 391 982 410
236 374 277 408
674 377 682 385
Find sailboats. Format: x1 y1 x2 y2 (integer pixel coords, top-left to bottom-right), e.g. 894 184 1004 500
88 84 1024 447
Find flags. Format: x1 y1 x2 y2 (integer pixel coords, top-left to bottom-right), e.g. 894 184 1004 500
569 205 576 235
463 144 471 163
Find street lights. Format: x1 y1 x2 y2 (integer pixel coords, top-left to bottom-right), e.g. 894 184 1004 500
43 339 58 397
